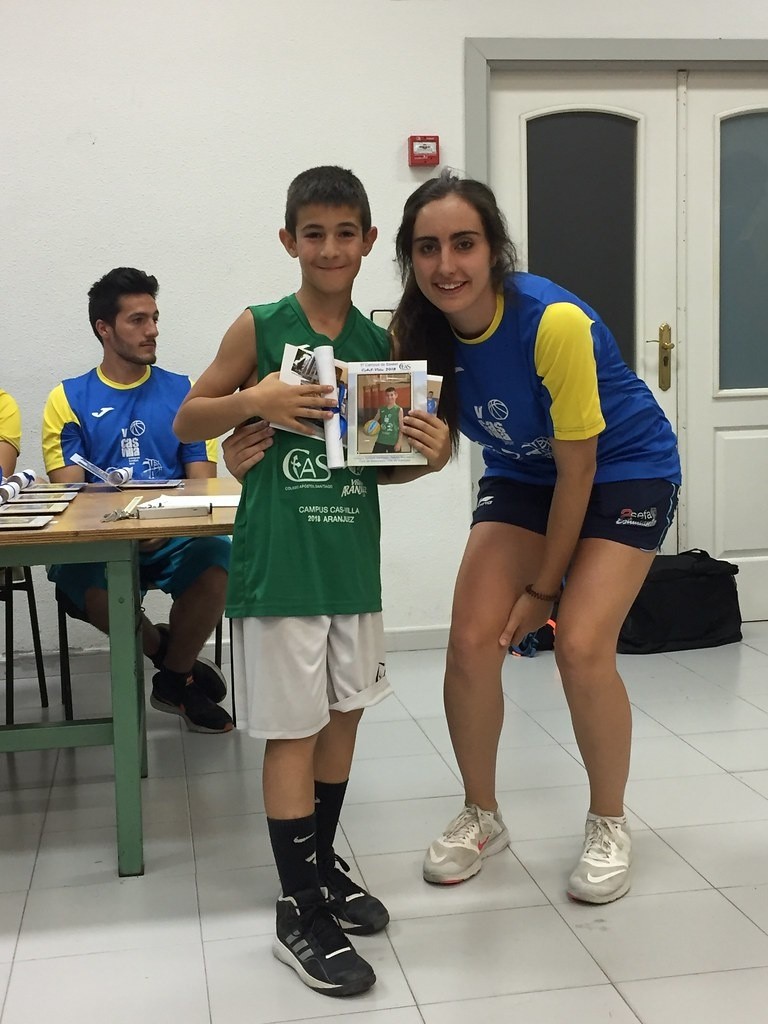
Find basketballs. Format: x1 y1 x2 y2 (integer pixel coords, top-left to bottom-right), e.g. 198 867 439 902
363 419 381 436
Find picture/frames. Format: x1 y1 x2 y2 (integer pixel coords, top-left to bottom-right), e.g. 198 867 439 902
346 360 429 466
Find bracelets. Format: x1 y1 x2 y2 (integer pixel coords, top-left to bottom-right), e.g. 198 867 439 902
524 582 563 603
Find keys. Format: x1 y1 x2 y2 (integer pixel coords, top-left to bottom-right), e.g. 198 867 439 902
99 508 131 523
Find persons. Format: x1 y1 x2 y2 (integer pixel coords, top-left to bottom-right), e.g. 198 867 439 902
0 391 21 588
220 168 684 903
363 387 403 453
425 390 436 413
172 166 452 996
43 267 235 736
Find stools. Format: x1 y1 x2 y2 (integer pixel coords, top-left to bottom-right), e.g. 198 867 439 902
0 566 237 727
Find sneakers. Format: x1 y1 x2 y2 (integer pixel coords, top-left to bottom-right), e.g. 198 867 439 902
273 888 376 995
423 801 510 884
151 624 227 702
316 845 389 935
150 671 233 734
567 814 632 903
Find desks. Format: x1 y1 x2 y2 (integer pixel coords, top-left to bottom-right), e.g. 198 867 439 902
0 478 243 879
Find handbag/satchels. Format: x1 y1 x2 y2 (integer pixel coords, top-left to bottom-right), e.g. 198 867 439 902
616 548 743 655
